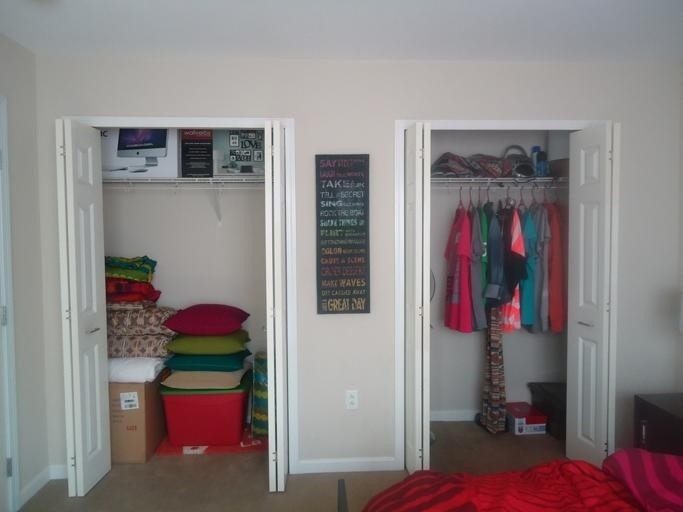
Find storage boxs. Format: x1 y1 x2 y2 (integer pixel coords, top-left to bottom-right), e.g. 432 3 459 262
504 401 549 436
108 368 173 465
160 373 252 447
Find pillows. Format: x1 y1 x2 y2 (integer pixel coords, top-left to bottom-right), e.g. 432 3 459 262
160 303 250 390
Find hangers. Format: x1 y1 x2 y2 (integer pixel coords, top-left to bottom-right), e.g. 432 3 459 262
455 181 564 212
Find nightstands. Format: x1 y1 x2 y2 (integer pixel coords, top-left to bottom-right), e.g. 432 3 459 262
634 394 682 454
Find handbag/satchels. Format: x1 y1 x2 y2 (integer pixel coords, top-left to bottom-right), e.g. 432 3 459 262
502 145 532 166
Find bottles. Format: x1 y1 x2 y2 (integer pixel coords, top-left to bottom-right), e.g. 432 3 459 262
531 146 547 177
